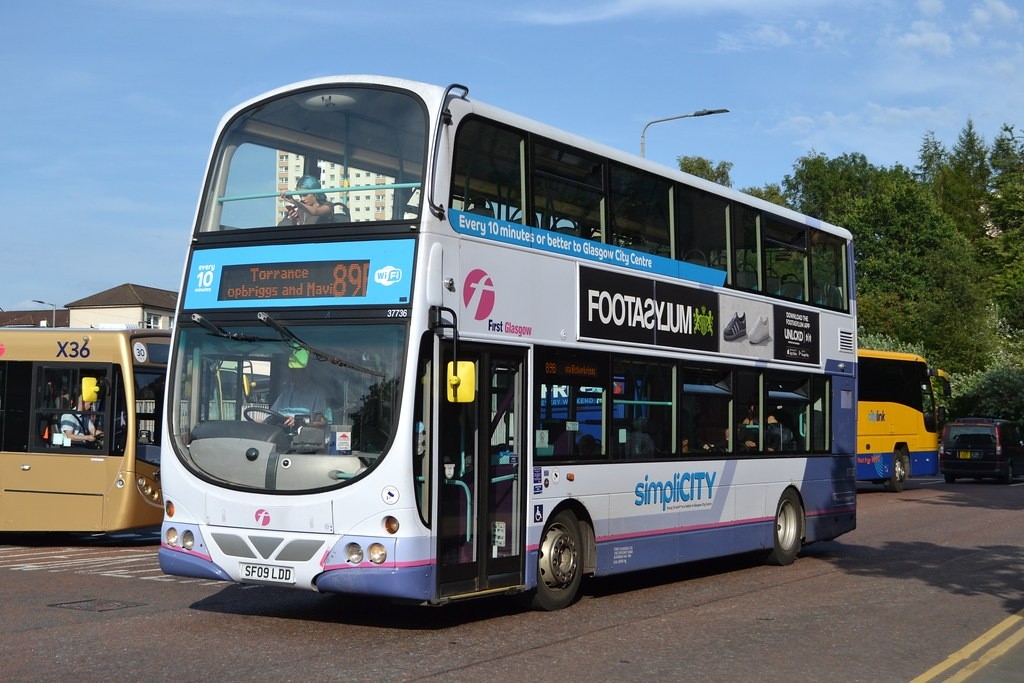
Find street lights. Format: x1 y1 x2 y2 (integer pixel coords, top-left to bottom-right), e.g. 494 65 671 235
33 300 56 327
641 109 730 160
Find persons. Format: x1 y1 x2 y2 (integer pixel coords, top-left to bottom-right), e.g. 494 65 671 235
578 434 601 457
255 355 333 444
61 394 104 442
278 175 334 226
682 400 793 453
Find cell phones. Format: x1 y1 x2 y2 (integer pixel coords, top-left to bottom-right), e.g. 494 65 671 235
286 206 300 218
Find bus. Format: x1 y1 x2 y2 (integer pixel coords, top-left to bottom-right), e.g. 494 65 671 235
0 324 256 531
159 75 858 610
539 348 951 493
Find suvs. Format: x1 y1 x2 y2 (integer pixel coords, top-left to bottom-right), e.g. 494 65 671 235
940 417 1024 484
